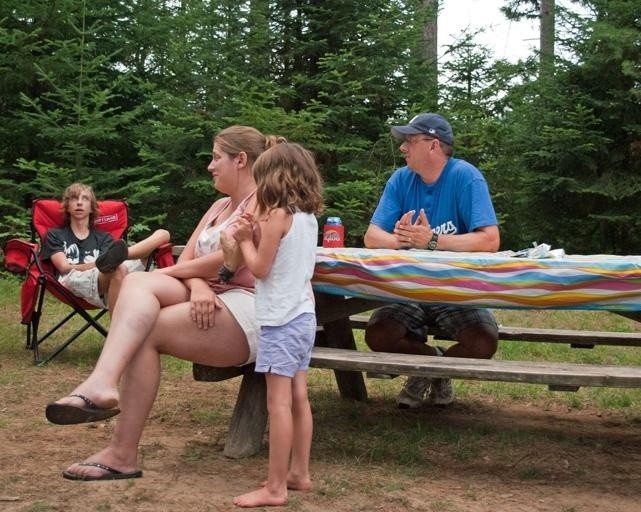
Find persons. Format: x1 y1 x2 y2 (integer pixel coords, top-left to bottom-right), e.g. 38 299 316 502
231 143 325 508
42 183 171 320
364 112 501 410
46 125 287 480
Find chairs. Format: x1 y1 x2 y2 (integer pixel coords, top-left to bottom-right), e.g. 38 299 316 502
3 200 175 366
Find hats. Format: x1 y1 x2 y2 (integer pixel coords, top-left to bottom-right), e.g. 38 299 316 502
391 113 453 146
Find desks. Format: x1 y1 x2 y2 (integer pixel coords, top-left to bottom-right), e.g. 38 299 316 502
193 248 641 460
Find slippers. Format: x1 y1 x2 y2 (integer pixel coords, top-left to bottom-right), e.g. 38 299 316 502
62 461 143 481
46 395 120 424
95 239 128 272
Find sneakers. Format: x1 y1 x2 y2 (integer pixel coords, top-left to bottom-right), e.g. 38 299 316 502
430 346 455 406
397 374 430 408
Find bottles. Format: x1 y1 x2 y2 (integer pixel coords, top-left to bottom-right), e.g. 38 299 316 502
323 216 345 248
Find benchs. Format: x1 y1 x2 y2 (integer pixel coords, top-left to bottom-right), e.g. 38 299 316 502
191 347 641 389
347 313 641 345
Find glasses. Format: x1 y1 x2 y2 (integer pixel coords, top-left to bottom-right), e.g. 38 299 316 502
404 136 433 144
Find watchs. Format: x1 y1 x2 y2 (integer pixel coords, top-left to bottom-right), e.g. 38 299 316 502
427 232 438 250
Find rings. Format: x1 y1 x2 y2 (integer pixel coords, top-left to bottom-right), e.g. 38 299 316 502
408 237 412 242
